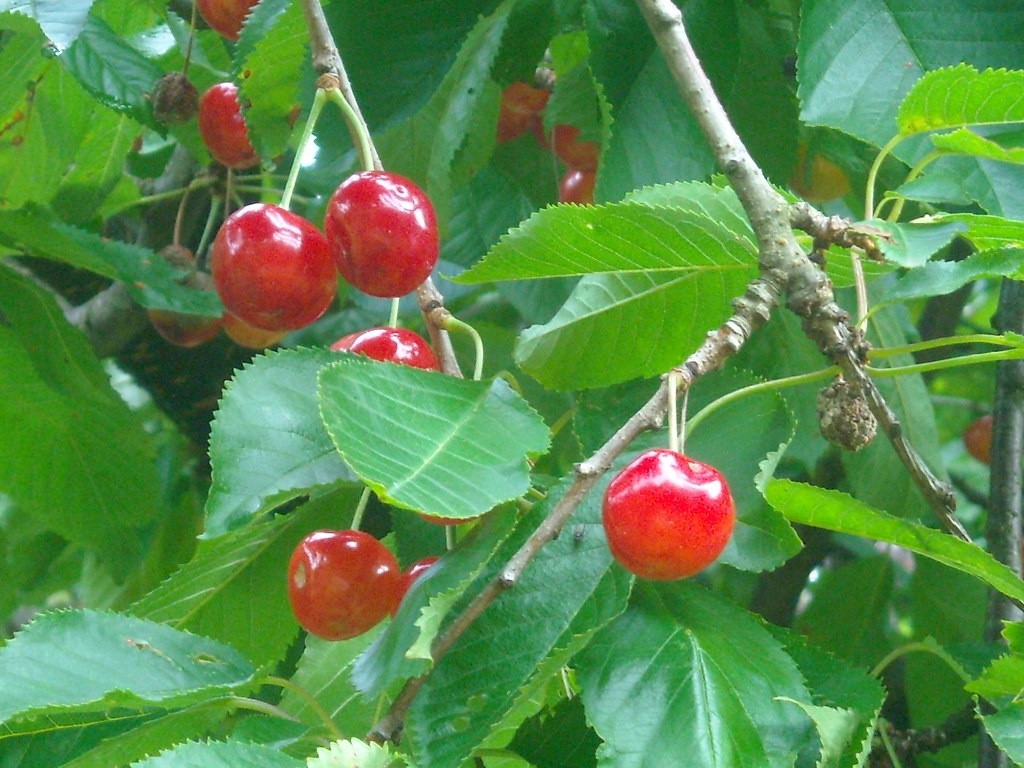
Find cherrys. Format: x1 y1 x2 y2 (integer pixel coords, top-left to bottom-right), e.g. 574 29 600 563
125 0 991 641
